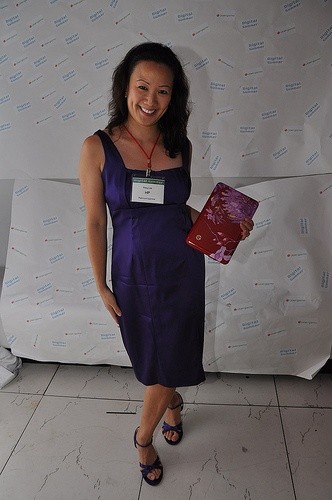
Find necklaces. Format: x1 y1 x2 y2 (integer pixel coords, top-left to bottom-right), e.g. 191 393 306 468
123 122 162 174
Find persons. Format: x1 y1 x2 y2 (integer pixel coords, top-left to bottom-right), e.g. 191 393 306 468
78 42 254 486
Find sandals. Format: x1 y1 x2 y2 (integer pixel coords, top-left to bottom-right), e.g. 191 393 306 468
132 425 163 486
161 391 184 446
